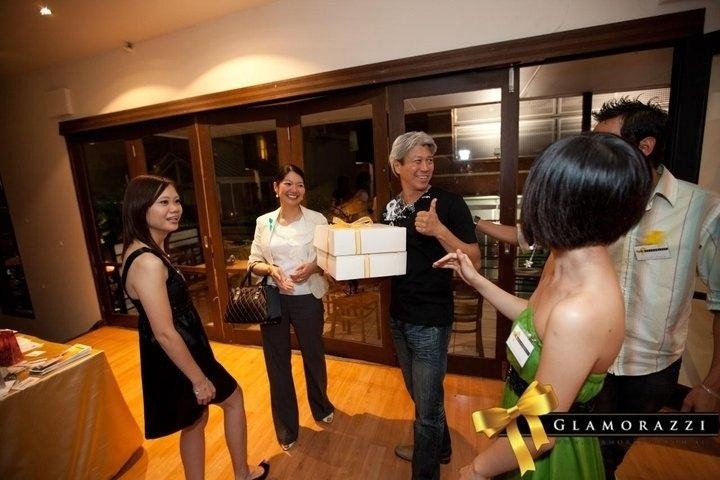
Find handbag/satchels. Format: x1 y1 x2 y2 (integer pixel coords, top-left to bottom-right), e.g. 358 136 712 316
223 260 281 324
0 329 26 367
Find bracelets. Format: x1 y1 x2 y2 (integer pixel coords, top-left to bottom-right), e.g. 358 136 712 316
192 376 210 395
473 215 481 228
696 380 719 401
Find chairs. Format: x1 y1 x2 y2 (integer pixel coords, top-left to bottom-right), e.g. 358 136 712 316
328 280 381 344
449 276 486 358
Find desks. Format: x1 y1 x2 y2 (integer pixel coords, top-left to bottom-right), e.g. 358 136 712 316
1 329 133 480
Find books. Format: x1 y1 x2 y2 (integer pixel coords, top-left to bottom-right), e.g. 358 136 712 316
29 342 93 378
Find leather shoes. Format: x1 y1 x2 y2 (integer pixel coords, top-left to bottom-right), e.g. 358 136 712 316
323 410 334 424
250 459 271 480
280 440 296 450
394 444 451 465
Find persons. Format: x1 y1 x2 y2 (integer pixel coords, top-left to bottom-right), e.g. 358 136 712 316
431 130 656 479
118 174 271 480
473 92 720 480
377 128 488 480
244 163 337 454
